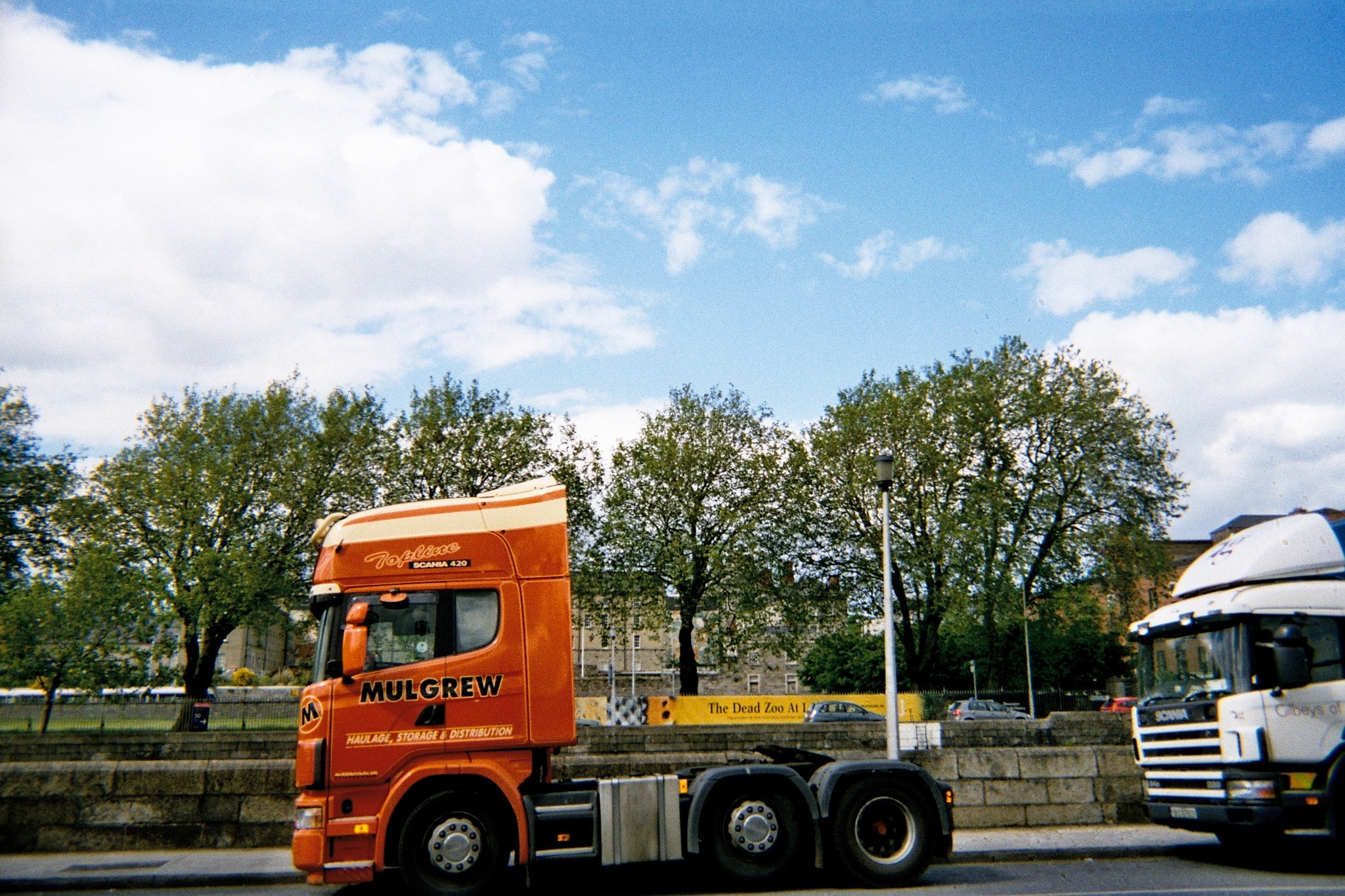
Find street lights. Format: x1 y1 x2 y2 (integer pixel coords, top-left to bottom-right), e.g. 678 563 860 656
872 449 905 762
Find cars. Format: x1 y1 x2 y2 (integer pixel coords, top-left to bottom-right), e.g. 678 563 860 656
804 701 886 723
946 697 1036 721
1099 697 1139 712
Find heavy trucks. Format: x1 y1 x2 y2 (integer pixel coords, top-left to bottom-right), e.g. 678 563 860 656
1125 512 1345 879
289 468 955 895
